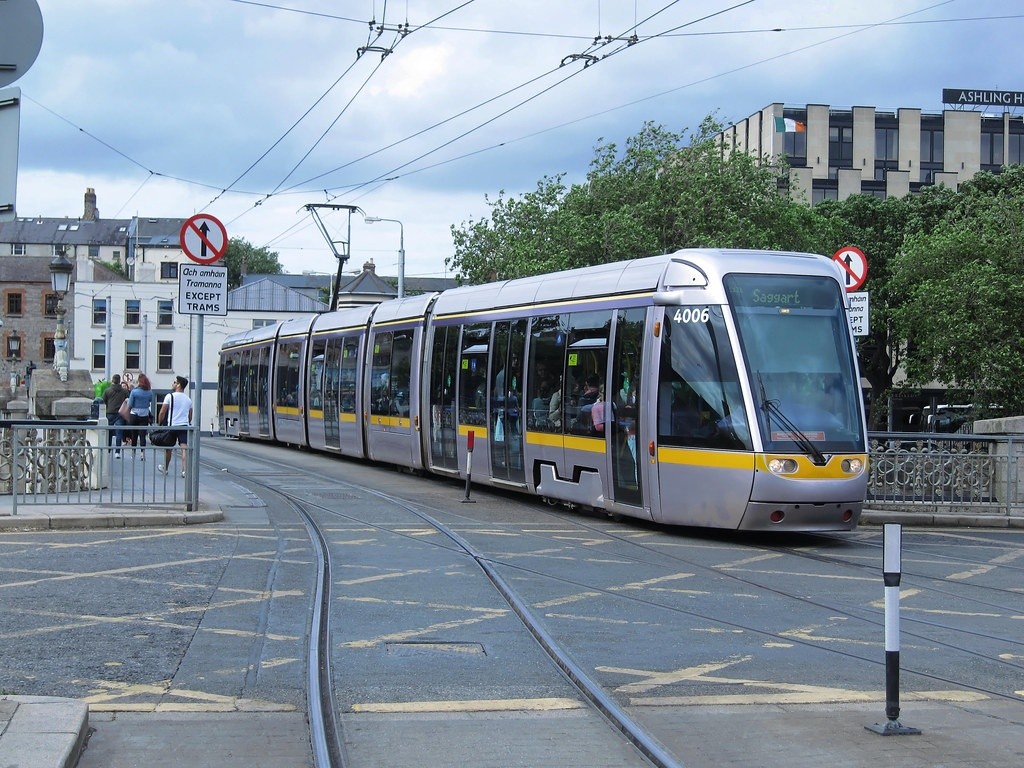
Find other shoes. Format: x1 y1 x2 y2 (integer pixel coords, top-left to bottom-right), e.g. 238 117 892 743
115 452 120 458
182 472 185 477
140 456 143 460
158 465 168 475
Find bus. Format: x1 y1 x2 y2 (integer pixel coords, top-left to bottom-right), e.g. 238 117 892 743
217 247 870 534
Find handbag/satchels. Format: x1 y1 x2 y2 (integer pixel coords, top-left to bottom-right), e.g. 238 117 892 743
149 430 176 447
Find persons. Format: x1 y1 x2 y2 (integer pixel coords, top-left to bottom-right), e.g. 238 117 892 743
99 373 153 461
223 345 624 436
155 376 194 479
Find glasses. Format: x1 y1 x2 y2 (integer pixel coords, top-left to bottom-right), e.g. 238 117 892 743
174 381 178 384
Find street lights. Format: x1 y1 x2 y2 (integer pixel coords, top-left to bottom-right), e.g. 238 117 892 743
364 217 405 298
7 330 22 401
47 249 75 380
